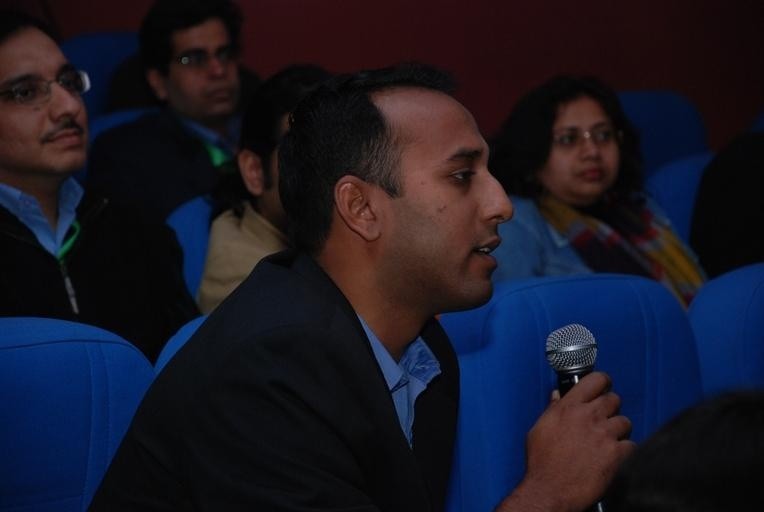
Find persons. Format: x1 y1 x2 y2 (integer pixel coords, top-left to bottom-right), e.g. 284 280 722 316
491 72 706 311
604 392 764 511
689 123 764 281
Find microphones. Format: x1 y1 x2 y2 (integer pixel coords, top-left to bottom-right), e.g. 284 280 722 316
545 322 613 512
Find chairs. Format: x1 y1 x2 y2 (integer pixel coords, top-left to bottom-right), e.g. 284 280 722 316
85 111 145 137
618 90 718 245
440 266 764 511
159 314 205 371
168 194 211 297
2 316 158 510
58 34 139 99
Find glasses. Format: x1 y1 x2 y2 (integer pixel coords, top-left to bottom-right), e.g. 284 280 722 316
0 69 90 110
